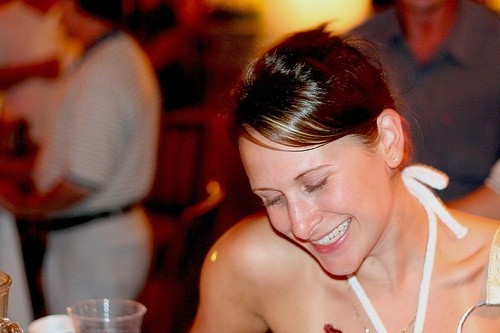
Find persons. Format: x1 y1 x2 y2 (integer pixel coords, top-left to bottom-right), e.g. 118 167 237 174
0 0 163 318
117 0 210 112
333 0 500 217
189 29 500 333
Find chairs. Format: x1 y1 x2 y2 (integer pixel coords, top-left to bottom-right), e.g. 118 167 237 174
150 119 224 333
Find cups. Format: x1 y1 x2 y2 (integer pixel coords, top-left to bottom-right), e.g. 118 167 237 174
0 271 12 323
66 298 147 333
27 314 74 333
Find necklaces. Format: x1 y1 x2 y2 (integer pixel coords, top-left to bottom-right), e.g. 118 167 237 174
344 274 424 333
